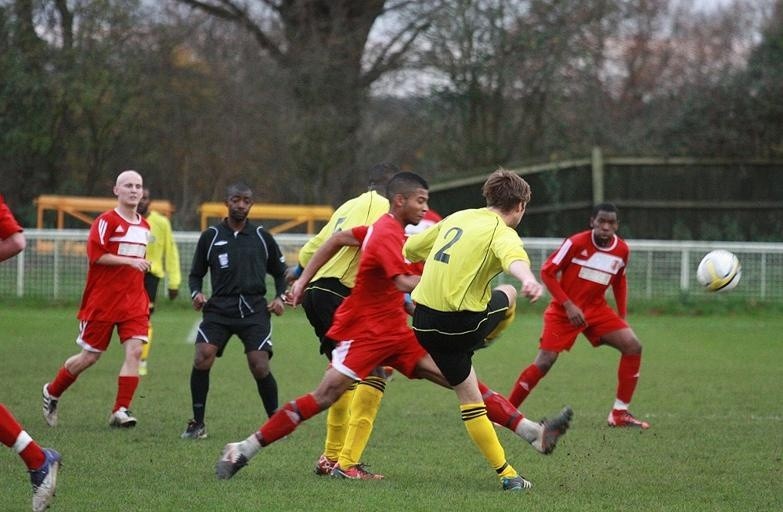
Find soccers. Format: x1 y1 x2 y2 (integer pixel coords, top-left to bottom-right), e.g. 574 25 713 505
695 249 744 294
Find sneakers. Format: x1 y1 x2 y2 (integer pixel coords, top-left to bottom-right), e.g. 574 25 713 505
216 443 249 480
607 408 650 430
108 411 139 429
530 408 574 454
313 456 383 481
30 448 62 512
502 474 532 492
180 420 207 440
42 383 59 428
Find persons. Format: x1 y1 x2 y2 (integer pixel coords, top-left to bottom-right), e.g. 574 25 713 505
403 166 544 492
0 196 62 511
176 183 288 442
42 168 151 432
216 157 574 486
493 203 653 430
137 190 178 374
